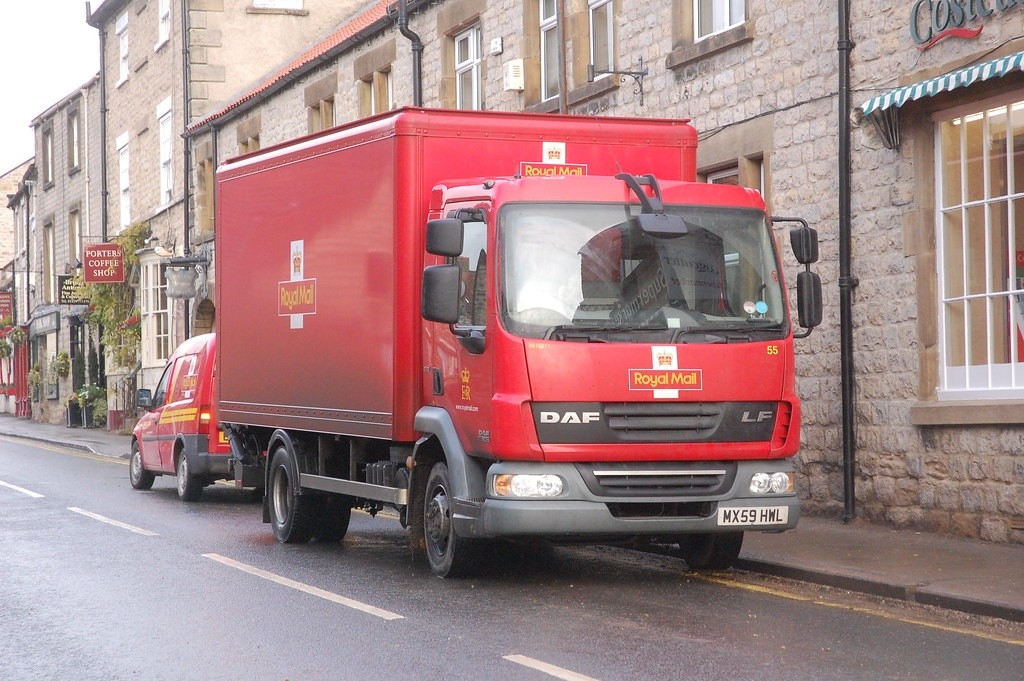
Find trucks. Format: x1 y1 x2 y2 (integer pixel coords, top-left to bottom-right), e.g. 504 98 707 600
206 102 827 582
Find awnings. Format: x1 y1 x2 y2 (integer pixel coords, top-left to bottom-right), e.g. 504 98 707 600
860 51 1024 149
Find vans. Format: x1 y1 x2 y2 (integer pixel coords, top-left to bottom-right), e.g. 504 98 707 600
127 332 269 502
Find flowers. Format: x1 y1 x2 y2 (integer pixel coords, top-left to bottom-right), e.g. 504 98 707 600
53 352 70 379
